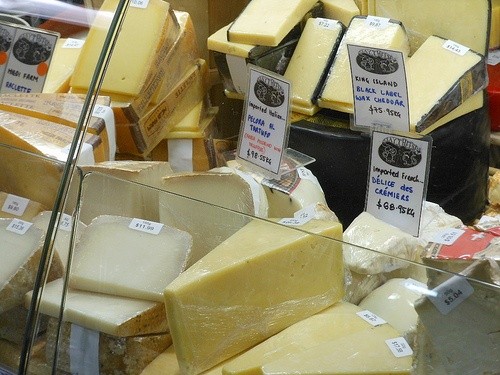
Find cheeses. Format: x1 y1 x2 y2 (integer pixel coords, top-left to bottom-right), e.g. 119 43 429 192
0 0 500 373
